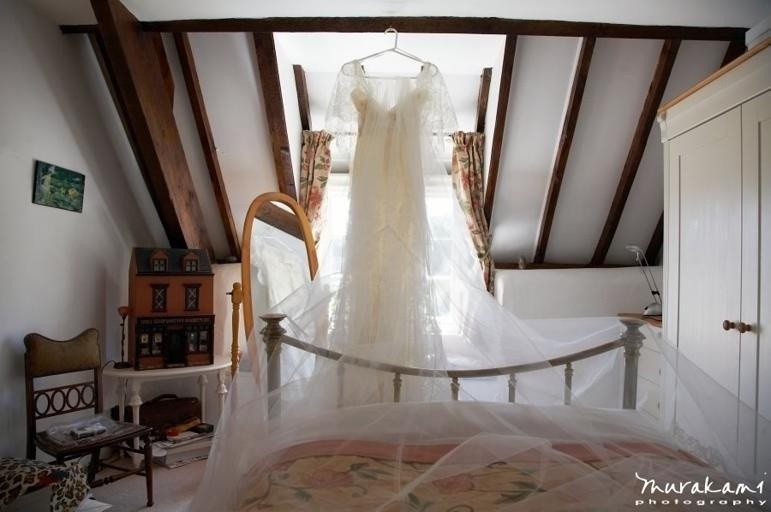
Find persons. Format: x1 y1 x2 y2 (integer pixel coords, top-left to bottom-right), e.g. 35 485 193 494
40 169 53 203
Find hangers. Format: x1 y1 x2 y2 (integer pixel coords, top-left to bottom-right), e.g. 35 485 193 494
339 27 438 81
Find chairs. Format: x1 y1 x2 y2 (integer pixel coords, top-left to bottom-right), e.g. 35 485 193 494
19 329 154 506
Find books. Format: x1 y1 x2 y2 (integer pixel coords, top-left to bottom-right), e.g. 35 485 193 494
152 429 213 468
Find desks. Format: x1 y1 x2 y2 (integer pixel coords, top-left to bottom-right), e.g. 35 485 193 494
102 362 236 467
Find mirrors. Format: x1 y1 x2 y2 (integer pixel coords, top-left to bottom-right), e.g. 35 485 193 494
240 191 324 402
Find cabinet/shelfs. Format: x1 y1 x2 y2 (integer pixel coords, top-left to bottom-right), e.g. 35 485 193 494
652 41 771 478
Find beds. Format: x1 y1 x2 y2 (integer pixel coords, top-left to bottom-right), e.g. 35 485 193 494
221 311 755 508
